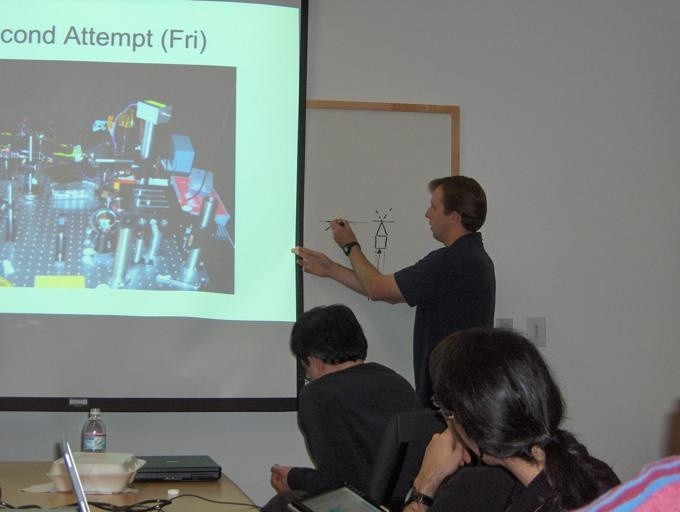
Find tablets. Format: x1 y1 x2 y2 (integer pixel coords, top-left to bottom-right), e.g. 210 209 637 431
288 480 396 512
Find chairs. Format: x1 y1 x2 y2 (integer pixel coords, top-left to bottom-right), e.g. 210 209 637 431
259 409 514 512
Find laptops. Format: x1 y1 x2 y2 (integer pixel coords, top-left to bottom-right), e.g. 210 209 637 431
132 455 222 482
60 435 91 512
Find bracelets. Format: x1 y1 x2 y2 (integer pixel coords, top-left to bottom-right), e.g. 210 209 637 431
403 485 436 507
342 241 363 257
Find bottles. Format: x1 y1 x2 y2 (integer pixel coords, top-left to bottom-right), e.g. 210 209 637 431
80 408 106 453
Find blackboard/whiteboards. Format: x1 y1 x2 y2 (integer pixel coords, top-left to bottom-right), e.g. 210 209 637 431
0 99 460 412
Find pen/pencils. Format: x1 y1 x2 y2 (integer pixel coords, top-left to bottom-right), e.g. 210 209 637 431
337 221 344 226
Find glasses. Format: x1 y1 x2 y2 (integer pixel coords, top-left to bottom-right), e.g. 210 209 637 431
430 392 444 408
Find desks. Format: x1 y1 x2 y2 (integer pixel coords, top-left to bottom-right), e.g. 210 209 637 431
0 461 259 512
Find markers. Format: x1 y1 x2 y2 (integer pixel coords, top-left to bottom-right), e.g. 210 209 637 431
64 399 100 405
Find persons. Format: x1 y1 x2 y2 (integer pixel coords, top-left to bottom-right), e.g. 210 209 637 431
291 177 499 426
267 302 429 511
394 326 627 512
